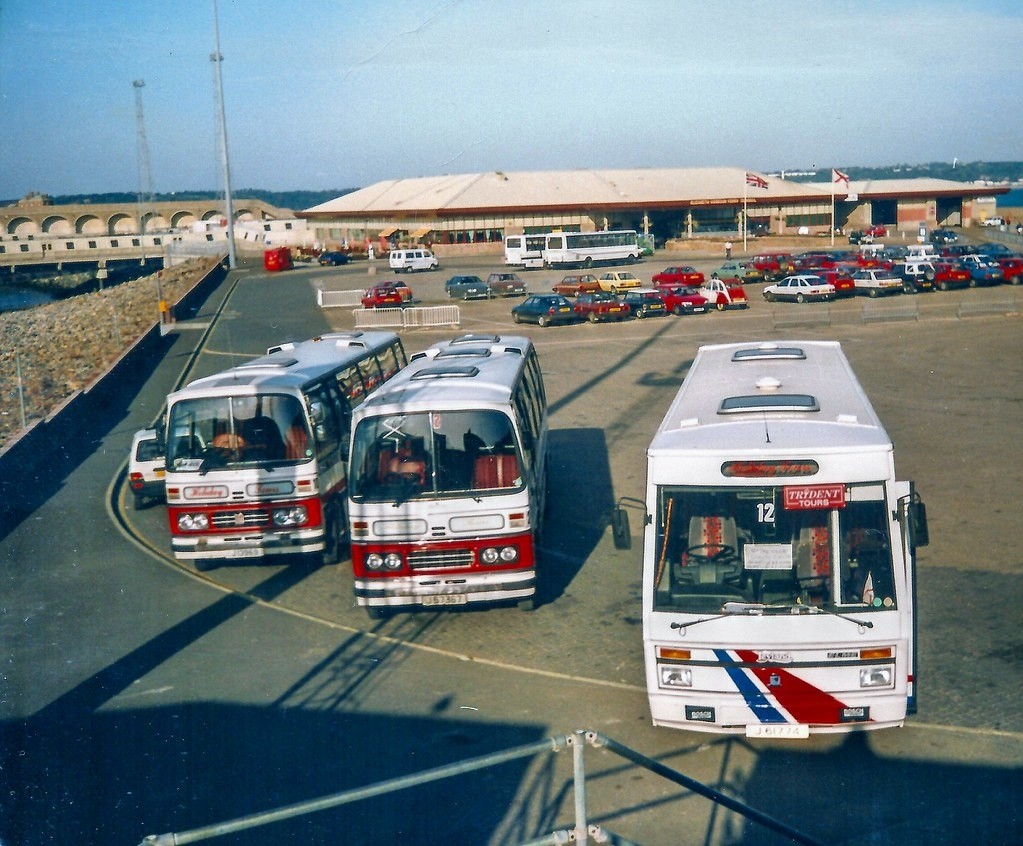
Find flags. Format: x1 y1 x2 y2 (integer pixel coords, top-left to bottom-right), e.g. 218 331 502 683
746 172 768 189
833 170 849 189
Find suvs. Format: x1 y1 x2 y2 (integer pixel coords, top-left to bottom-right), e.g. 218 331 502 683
750 253 796 281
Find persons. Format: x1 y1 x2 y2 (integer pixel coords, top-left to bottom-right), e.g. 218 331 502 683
1001 217 1005 231
1016 222 1023 235
725 240 732 259
1006 218 1010 230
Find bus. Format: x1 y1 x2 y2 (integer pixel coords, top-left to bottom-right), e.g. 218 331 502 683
156 334 408 569
609 342 930 737
342 333 546 617
545 230 638 267
504 233 547 266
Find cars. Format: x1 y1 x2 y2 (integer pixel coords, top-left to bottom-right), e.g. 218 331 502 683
488 272 526 297
552 267 747 323
979 216 1004 227
930 229 957 245
360 279 413 309
751 226 776 238
638 246 648 258
318 251 352 267
847 230 873 245
865 225 887 237
511 293 579 328
446 275 493 301
712 242 1023 303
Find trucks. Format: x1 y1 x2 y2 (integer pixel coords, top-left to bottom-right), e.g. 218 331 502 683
265 248 294 271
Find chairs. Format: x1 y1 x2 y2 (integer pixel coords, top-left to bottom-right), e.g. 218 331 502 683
377 440 423 492
284 424 308 458
212 435 244 462
797 517 850 592
680 511 742 585
474 453 519 488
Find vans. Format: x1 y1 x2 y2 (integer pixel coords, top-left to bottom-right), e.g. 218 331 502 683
129 428 209 510
389 249 439 274
